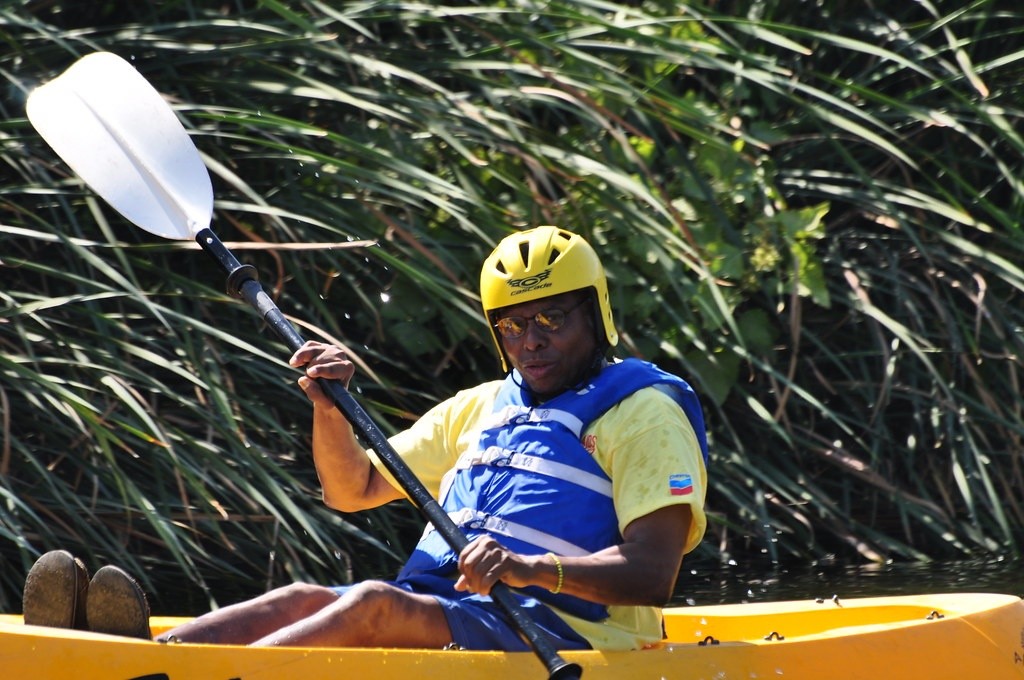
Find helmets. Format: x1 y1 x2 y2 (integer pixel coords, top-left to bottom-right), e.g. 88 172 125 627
480 226 619 373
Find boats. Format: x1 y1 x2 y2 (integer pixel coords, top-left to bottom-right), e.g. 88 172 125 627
0 592 1024 680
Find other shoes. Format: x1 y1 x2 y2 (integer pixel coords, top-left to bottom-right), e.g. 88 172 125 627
22 550 89 630
86 565 154 641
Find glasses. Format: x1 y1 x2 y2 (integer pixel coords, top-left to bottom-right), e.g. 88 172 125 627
494 296 589 339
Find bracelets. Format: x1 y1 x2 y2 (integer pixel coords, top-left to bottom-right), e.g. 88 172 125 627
546 552 563 594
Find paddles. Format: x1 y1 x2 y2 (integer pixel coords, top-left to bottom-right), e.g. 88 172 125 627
25 51 583 680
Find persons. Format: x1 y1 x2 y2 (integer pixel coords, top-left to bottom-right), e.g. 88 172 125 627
22 227 712 653
